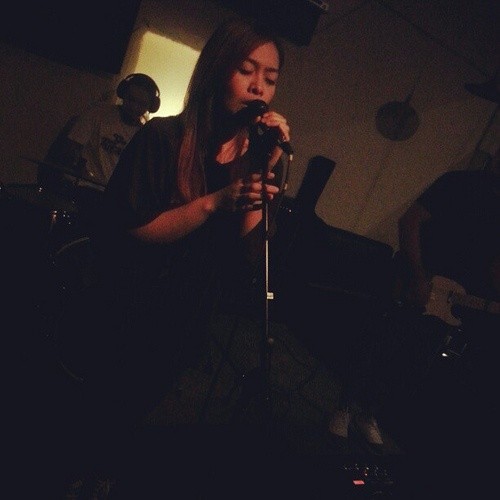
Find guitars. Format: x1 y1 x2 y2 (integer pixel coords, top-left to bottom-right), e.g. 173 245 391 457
391 275 500 326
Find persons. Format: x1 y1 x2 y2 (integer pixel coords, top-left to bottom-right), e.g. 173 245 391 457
331 145 500 445
56 17 289 500
50 74 160 185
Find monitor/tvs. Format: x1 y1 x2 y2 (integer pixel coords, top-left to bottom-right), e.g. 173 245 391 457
0 0 141 76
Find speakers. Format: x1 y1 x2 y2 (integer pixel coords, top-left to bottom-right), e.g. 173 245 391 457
230 0 322 45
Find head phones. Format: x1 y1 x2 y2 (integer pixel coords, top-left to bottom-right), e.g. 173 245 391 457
117 74 160 113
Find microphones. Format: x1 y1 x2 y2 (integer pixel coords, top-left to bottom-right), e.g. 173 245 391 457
246 99 294 155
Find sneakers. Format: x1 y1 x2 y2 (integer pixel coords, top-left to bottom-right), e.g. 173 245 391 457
356 414 384 445
329 410 352 438
